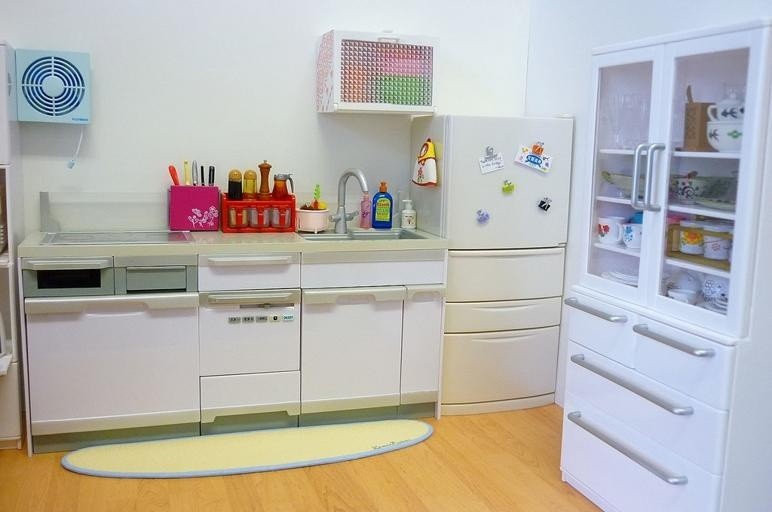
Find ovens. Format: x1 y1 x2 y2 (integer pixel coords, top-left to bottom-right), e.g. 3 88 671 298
198 289 301 375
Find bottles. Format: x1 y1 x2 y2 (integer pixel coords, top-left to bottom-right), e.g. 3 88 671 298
227 169 243 200
243 168 256 200
272 173 294 198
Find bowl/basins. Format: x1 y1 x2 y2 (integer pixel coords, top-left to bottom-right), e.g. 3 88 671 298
706 119 744 152
621 223 645 249
671 173 734 204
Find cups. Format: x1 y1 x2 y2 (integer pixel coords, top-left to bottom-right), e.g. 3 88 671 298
678 218 734 262
598 214 625 242
668 289 698 309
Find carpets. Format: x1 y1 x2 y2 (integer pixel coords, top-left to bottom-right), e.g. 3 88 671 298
61 419 432 478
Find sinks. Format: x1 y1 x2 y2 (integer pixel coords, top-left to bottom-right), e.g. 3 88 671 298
301 230 428 242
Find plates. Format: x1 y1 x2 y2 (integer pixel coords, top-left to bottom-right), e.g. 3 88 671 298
695 198 738 209
608 268 670 286
702 277 728 313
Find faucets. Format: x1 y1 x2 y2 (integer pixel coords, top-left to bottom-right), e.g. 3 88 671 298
329 167 368 234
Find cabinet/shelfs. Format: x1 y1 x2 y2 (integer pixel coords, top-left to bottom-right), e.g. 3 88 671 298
559 18 772 512
0 41 23 450
19 250 448 457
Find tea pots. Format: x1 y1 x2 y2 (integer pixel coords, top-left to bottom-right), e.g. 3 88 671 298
707 92 745 120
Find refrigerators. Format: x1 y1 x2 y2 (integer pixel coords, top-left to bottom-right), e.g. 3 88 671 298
409 115 575 416
